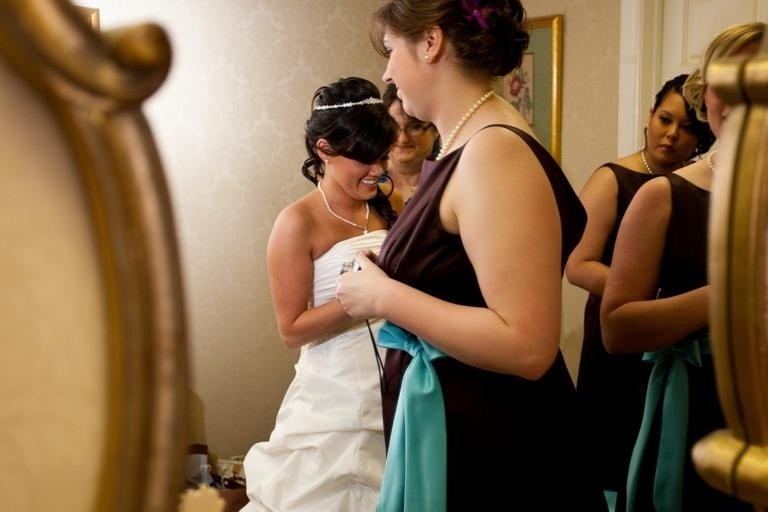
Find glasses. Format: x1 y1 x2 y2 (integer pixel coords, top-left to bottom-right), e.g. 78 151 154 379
397 121 432 137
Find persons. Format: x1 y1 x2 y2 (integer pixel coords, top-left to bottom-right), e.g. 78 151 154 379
335 0 609 512
236 78 407 511
600 23 766 511
564 75 716 494
380 83 440 200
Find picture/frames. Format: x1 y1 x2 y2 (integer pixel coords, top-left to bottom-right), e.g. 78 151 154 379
491 14 564 167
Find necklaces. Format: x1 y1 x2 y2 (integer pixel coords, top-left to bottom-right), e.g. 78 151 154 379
318 178 371 235
639 150 656 178
411 183 418 195
431 89 498 162
709 148 718 174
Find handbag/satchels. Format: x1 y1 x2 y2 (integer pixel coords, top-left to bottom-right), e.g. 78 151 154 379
179 464 249 512
